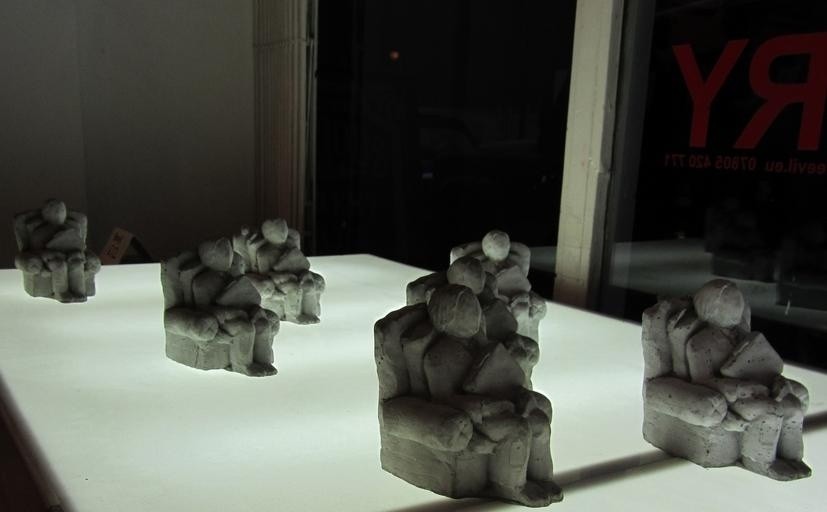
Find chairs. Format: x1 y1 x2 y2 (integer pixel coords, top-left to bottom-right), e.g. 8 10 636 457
159 248 280 371
232 217 325 320
640 295 810 469
11 210 103 298
